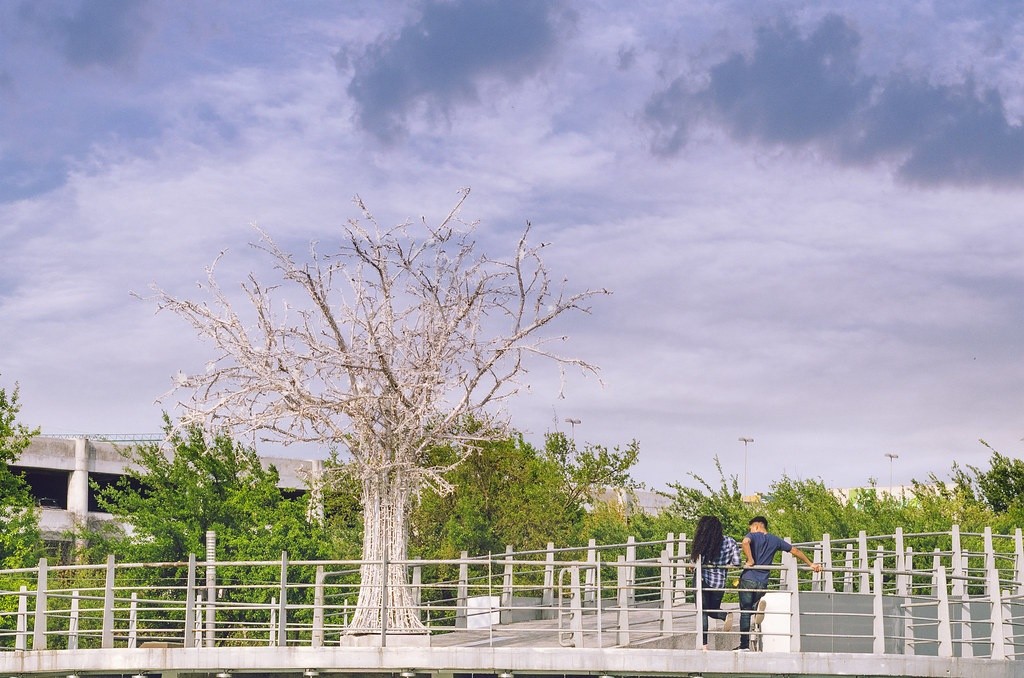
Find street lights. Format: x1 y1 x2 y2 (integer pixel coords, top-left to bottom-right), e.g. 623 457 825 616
738 436 754 500
885 452 899 501
564 416 581 459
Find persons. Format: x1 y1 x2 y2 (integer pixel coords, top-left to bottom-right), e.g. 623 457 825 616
733 517 823 651
689 516 742 651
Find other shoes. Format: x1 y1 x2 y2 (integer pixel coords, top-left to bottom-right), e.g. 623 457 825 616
731 646 750 652
755 599 767 624
724 612 733 632
703 645 708 650
